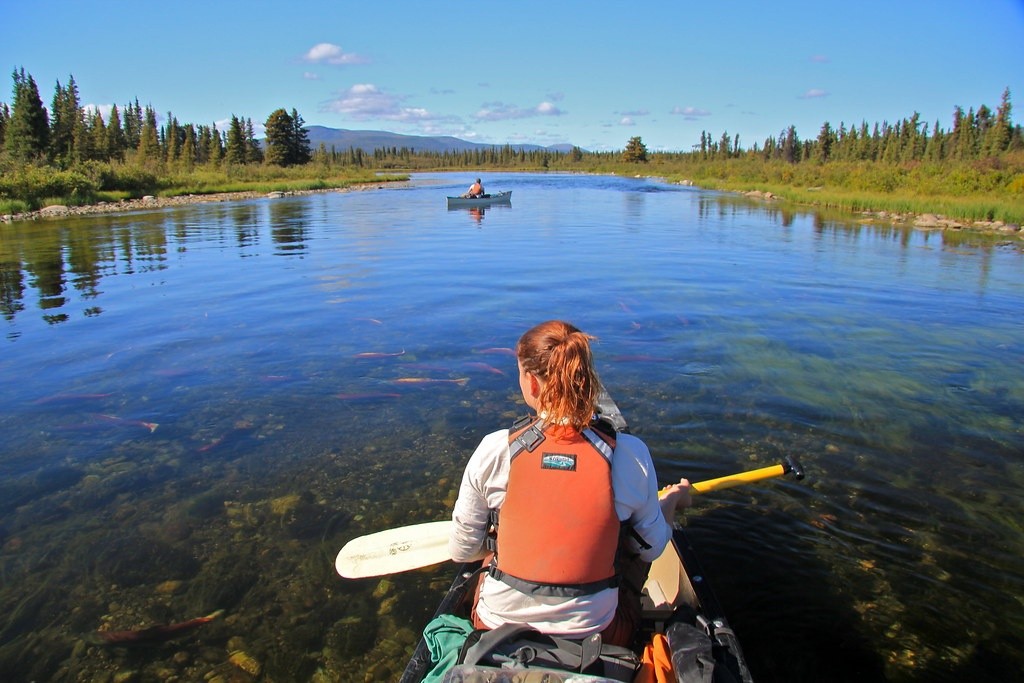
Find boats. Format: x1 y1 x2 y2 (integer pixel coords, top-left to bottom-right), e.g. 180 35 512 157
445 190 513 206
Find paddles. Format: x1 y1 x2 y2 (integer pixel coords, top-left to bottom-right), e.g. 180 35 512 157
333 452 806 581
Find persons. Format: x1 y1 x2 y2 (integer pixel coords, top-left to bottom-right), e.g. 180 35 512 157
448 320 694 648
468 178 485 199
